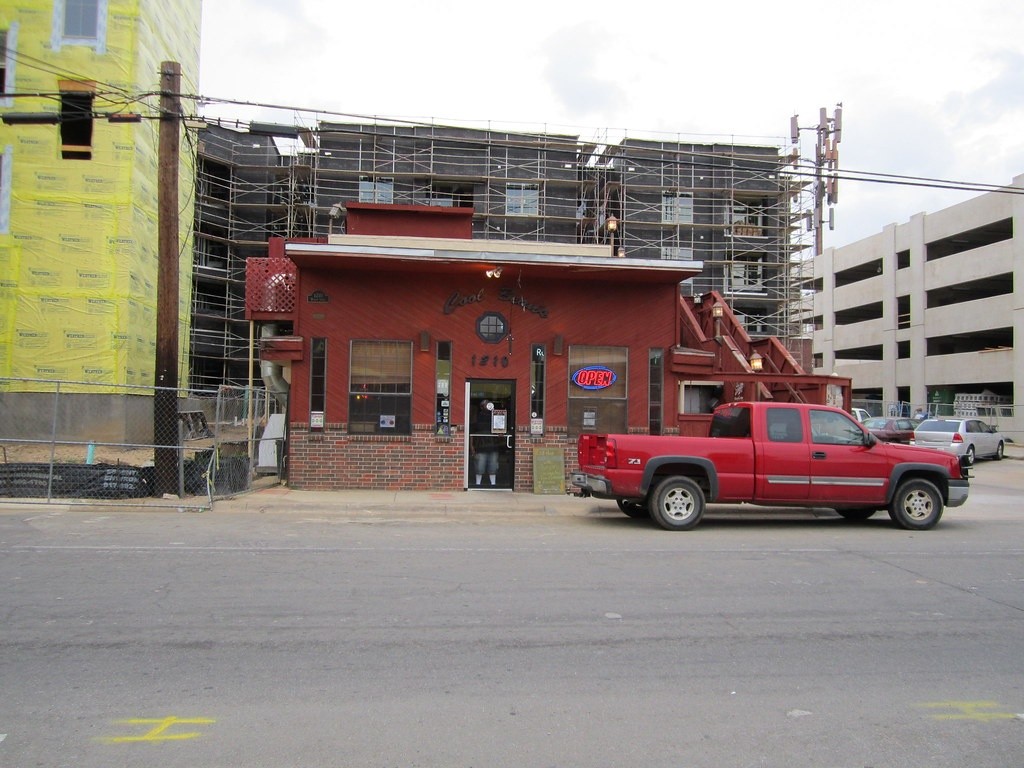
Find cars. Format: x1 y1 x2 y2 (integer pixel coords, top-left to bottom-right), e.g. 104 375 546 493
910 418 1005 466
852 408 923 445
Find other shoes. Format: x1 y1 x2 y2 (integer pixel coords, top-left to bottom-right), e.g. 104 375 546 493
490 485 496 488
475 484 480 488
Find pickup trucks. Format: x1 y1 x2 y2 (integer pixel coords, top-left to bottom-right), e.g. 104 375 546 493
570 402 975 531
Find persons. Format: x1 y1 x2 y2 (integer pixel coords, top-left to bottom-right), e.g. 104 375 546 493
470 399 500 486
